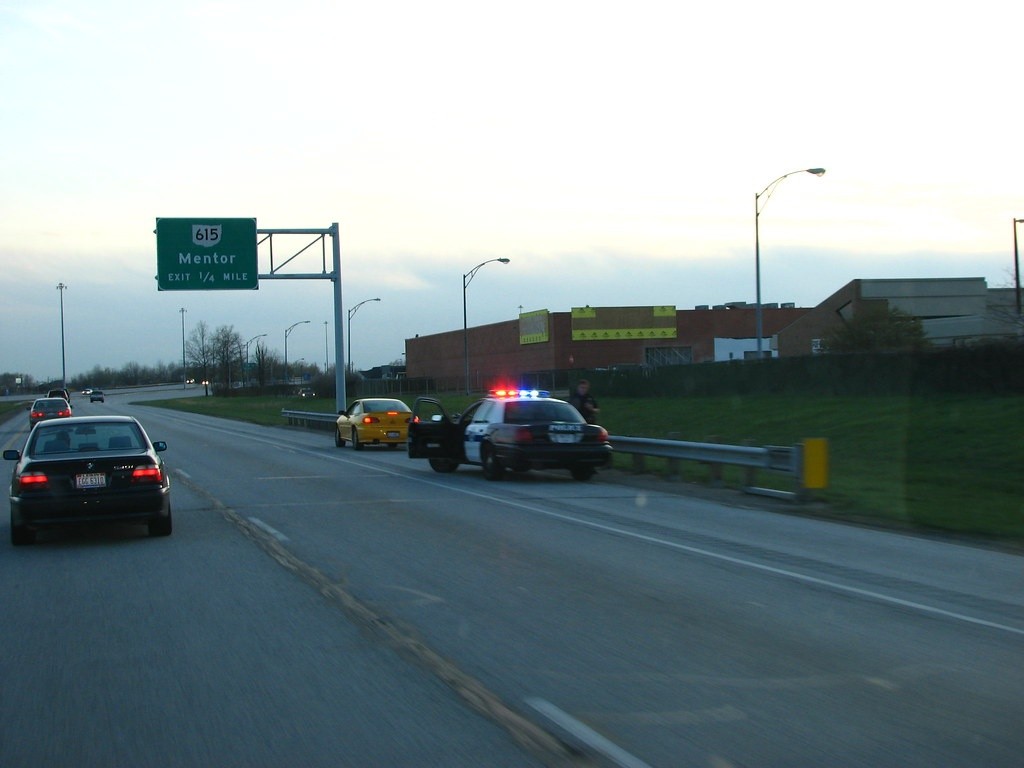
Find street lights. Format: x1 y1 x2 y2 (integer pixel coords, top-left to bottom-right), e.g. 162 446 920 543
462 257 510 395
755 169 828 358
347 298 382 373
1013 217 1023 318
179 307 187 390
245 334 268 387
284 320 312 383
324 320 328 373
55 282 68 386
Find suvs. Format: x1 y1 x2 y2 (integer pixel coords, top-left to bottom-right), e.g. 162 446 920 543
45 389 70 404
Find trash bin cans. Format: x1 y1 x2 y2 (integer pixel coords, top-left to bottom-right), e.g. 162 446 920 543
803 437 827 491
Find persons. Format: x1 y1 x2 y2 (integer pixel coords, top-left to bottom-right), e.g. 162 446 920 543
53 431 70 450
567 380 601 425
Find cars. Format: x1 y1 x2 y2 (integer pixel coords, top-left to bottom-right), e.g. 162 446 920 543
83 388 94 395
3 415 172 544
89 390 106 402
407 388 614 480
335 398 412 450
26 397 73 430
